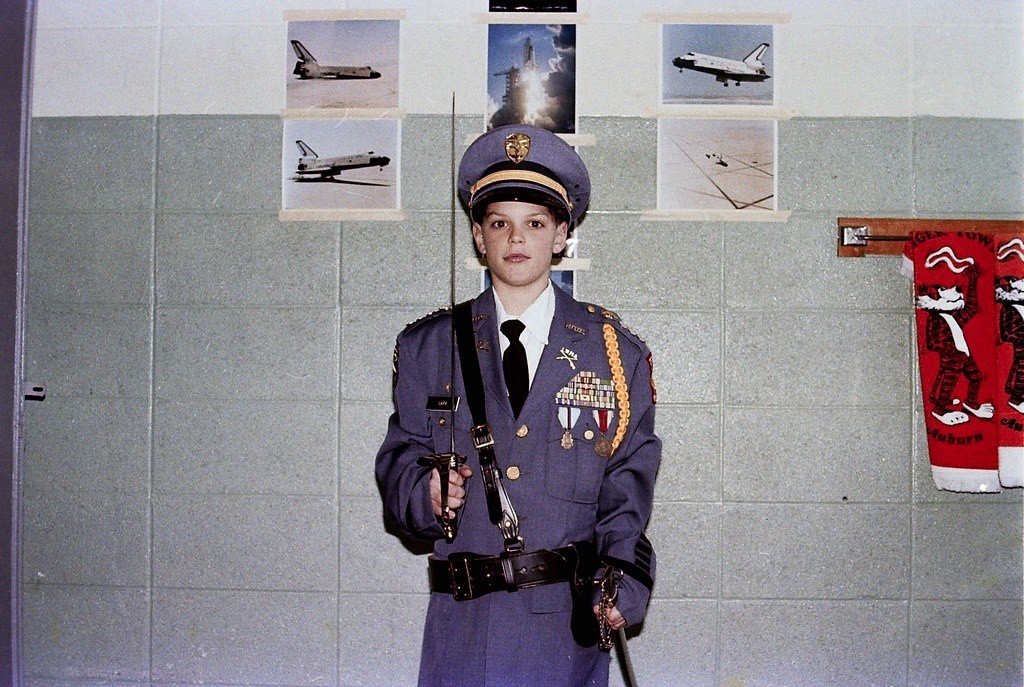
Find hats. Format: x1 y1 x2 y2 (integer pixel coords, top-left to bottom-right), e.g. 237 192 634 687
459 124 591 228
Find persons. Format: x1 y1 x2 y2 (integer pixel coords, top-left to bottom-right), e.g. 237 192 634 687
375 124 662 687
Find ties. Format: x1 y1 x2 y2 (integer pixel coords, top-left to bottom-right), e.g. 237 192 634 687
500 319 530 421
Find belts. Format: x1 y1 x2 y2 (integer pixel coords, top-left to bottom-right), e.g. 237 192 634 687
427 544 597 602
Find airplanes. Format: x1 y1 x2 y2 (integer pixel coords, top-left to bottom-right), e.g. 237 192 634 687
295 139 392 181
290 40 382 81
672 41 772 87
